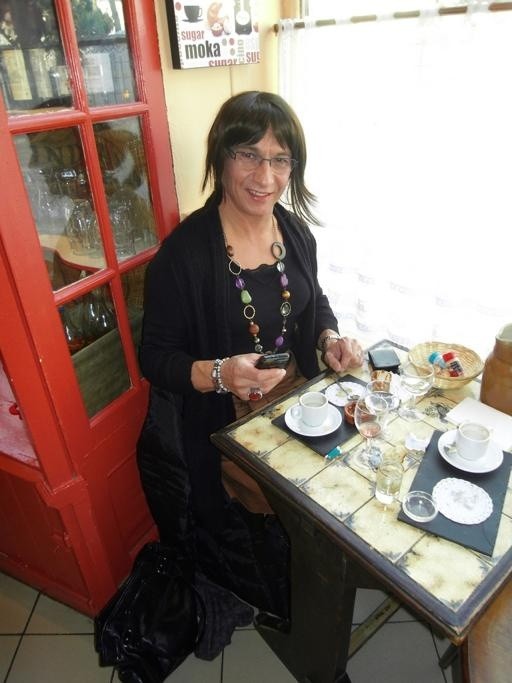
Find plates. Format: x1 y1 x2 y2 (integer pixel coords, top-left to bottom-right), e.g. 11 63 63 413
431 476 495 527
284 401 345 438
326 380 365 406
181 18 204 22
436 428 504 474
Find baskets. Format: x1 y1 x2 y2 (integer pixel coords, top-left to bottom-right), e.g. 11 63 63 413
408 342 485 388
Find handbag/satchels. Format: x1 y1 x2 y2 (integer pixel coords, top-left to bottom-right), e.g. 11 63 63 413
94 541 205 683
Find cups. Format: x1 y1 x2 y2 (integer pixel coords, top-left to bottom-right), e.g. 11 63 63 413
292 391 329 425
405 425 429 449
369 369 392 391
182 3 203 21
374 460 403 503
454 420 492 461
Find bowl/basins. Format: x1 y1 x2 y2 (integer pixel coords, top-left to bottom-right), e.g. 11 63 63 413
399 490 439 524
345 402 370 425
211 23 224 35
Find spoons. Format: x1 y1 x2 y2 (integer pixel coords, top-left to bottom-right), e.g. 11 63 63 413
330 374 359 400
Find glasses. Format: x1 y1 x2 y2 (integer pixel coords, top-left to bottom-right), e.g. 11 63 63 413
223 146 299 175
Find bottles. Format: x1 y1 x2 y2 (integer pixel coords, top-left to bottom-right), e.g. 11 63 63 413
59 270 146 355
19 162 148 256
0 0 135 110
235 0 253 34
427 350 449 371
443 350 464 376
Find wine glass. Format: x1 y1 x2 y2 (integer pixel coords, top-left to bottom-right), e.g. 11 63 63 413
394 359 435 424
353 381 400 470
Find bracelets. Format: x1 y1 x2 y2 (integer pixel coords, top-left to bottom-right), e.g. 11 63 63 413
211 357 231 396
321 335 341 351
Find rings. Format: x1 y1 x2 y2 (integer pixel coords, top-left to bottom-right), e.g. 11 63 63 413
248 388 263 402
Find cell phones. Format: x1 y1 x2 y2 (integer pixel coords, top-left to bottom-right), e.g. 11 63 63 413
258 352 290 370
369 348 400 370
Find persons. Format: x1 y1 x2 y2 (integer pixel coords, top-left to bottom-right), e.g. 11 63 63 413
136 91 363 620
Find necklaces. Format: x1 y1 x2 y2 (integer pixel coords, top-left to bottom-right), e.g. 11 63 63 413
221 215 292 355
